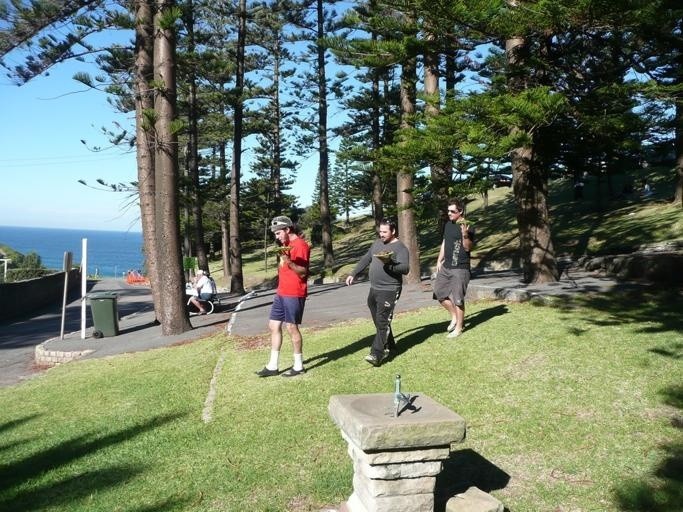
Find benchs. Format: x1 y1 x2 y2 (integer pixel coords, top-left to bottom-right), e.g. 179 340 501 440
191 293 220 316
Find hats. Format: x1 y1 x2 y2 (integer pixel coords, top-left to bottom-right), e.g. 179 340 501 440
269 215 294 234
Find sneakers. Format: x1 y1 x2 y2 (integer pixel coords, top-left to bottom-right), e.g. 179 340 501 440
280 365 306 377
196 310 207 316
446 327 463 339
446 318 458 332
378 343 398 364
252 365 279 378
363 352 382 368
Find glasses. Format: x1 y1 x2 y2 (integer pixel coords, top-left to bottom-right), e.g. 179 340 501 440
270 221 287 227
447 209 460 214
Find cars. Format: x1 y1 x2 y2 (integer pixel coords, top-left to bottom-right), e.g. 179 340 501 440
488 174 512 190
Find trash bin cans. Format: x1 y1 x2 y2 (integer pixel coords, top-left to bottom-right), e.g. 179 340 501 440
85 291 119 339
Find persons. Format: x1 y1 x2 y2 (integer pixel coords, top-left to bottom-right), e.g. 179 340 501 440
191 270 217 315
254 216 310 377
346 218 409 367
432 198 474 339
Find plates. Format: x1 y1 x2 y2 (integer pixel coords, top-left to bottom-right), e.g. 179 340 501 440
374 250 395 258
271 246 293 252
455 220 475 225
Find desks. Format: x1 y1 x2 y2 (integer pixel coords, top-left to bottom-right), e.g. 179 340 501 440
185 287 195 296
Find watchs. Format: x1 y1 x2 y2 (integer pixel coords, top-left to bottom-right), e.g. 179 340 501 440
463 235 469 239
